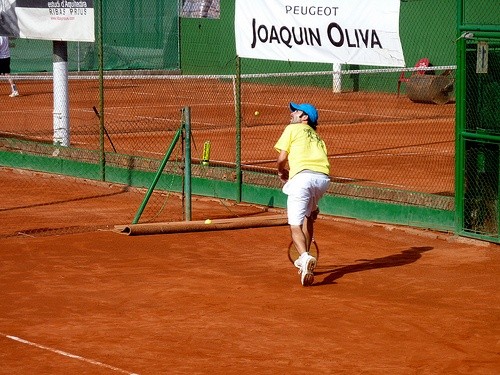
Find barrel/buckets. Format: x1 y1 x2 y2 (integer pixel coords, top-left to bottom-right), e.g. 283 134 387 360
407 75 455 105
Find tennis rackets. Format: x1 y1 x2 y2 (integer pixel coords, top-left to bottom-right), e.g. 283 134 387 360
288 218 319 269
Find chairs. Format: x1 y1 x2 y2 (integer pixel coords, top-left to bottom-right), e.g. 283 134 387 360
396 58 430 99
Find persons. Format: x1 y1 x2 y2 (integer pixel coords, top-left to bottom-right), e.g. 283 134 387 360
0 36 19 98
274 102 330 288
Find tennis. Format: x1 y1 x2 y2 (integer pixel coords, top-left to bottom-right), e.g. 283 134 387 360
204 219 212 224
255 111 260 115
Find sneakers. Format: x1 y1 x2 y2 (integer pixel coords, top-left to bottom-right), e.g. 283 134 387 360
294 252 309 268
9 91 19 97
301 257 315 286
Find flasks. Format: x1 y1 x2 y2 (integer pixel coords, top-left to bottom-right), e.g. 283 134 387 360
202 141 210 166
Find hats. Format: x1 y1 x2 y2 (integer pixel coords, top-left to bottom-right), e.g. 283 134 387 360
290 101 319 123
